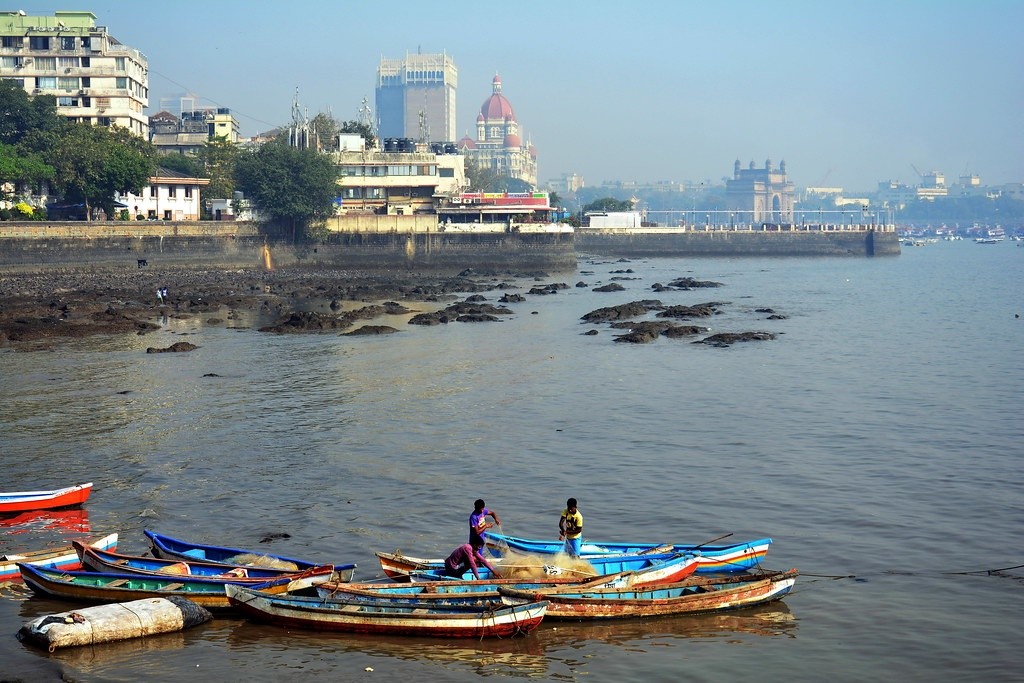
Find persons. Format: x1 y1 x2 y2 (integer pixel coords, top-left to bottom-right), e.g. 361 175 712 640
445 536 503 580
559 498 583 559
469 499 501 555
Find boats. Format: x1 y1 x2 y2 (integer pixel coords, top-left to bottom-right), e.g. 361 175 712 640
143 529 357 584
483 530 773 574
310 549 702 604
70 540 335 593
15 562 292 613
894 222 1024 247
0 532 119 582
496 567 802 620
224 582 552 639
0 483 94 513
15 595 214 654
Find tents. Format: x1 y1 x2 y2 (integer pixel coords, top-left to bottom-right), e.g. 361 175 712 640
45 189 128 221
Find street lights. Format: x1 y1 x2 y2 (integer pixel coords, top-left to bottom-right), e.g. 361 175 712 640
731 213 734 230
802 214 805 230
850 214 854 225
706 214 709 225
681 213 685 226
